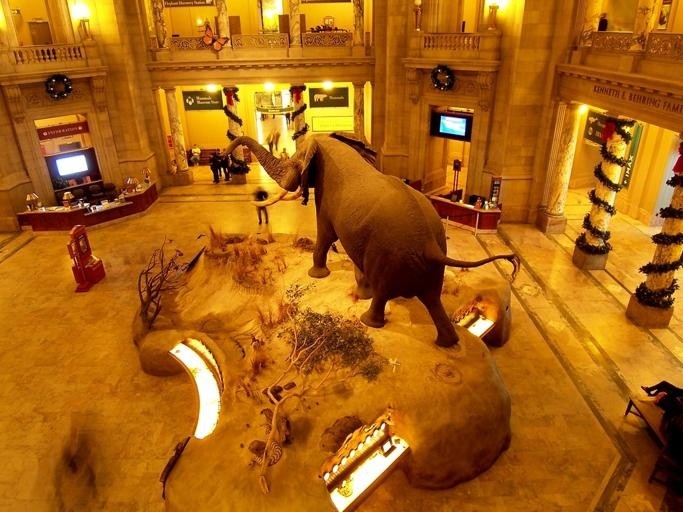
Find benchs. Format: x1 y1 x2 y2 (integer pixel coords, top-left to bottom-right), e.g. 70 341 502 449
191 148 251 164
623 395 666 448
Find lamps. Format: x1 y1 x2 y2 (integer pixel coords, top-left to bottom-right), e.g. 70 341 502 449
63 191 76 209
141 167 152 184
127 176 140 192
26 192 39 210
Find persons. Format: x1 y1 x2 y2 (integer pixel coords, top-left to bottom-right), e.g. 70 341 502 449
596 13 607 33
273 130 280 151
208 152 219 183
284 105 291 127
639 379 683 398
222 147 230 182
189 144 201 167
214 149 222 177
277 147 288 159
250 185 270 225
265 131 273 154
39 41 57 63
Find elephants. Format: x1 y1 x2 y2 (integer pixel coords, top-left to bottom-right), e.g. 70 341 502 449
221 126 518 344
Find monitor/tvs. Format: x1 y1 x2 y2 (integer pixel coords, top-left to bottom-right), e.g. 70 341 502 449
439 115 467 137
56 154 88 177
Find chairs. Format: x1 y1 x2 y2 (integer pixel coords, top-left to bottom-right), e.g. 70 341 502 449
70 183 117 204
